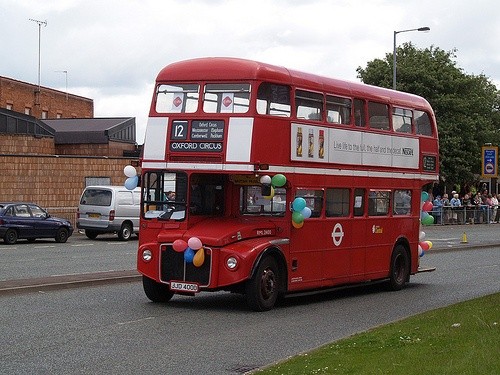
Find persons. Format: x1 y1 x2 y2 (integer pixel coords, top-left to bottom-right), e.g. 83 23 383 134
433 189 500 224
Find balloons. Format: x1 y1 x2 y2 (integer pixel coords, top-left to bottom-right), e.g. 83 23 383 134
184 248 194 263
188 236 202 250
421 191 435 225
193 247 205 267
124 176 138 190
418 232 432 258
263 185 275 200
292 198 311 229
124 165 137 178
260 174 271 186
272 173 287 187
173 240 189 253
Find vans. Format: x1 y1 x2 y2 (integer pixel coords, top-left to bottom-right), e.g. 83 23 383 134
76 185 141 241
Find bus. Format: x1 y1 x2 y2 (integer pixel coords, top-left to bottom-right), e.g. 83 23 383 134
137 58 440 312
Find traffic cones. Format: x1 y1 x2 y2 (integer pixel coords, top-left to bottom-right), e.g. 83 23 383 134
460 232 469 243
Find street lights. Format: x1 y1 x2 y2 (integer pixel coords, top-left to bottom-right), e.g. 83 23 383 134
393 26 431 93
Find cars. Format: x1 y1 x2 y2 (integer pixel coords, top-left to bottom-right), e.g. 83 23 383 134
0 203 74 245
